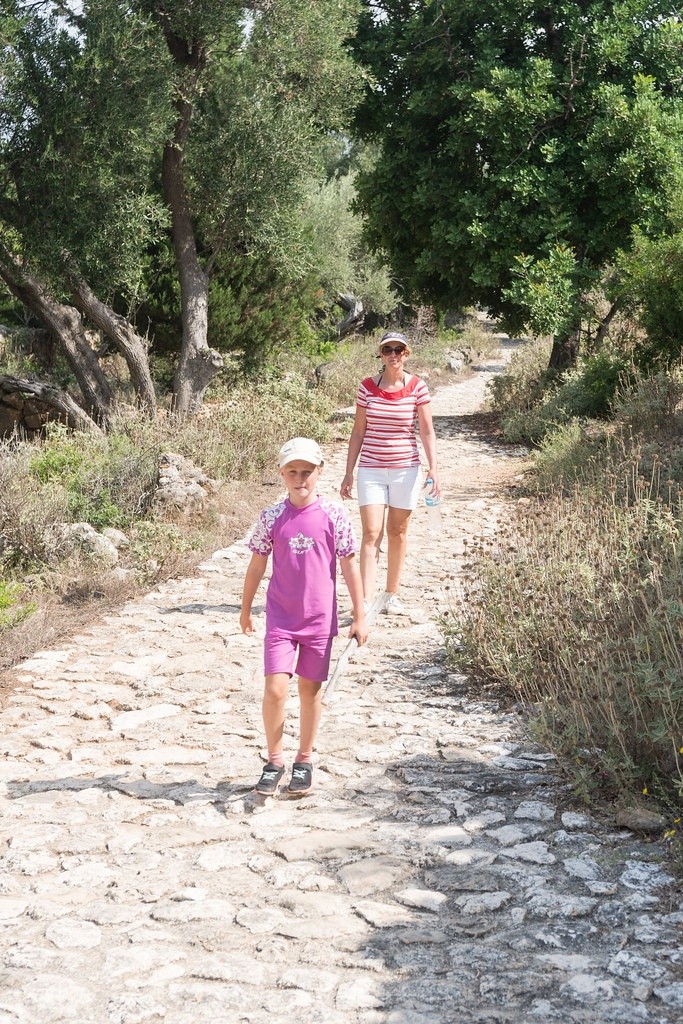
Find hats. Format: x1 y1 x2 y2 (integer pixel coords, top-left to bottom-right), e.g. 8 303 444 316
379 331 408 349
278 437 322 468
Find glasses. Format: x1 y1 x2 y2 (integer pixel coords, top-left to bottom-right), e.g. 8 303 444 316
381 346 405 356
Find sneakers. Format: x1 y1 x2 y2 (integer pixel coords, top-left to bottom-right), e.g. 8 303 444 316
386 595 404 615
288 762 313 793
255 762 287 796
364 599 373 616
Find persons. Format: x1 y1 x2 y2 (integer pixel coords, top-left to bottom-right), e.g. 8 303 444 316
339 331 442 616
238 437 368 797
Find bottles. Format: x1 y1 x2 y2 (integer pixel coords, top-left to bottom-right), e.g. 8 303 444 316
425 478 443 530
